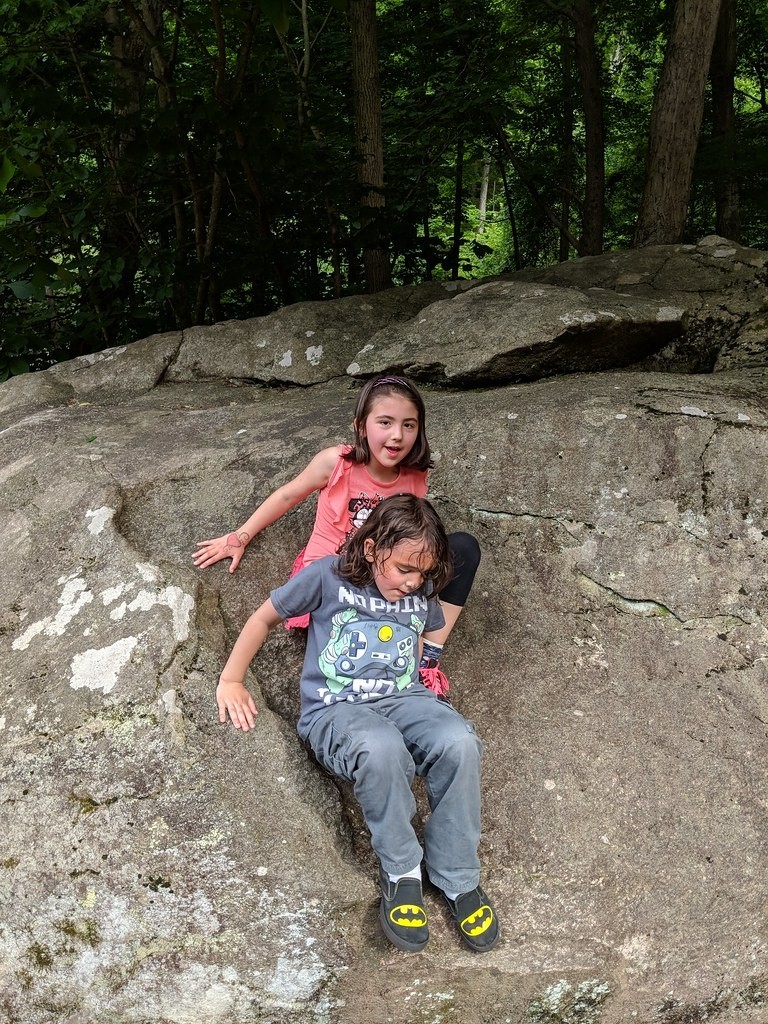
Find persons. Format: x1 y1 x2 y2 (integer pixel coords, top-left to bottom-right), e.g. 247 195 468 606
192 375 481 709
218 493 501 955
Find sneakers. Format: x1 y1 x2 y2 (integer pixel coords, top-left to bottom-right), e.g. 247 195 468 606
379 863 428 952
441 884 500 952
418 657 451 706
